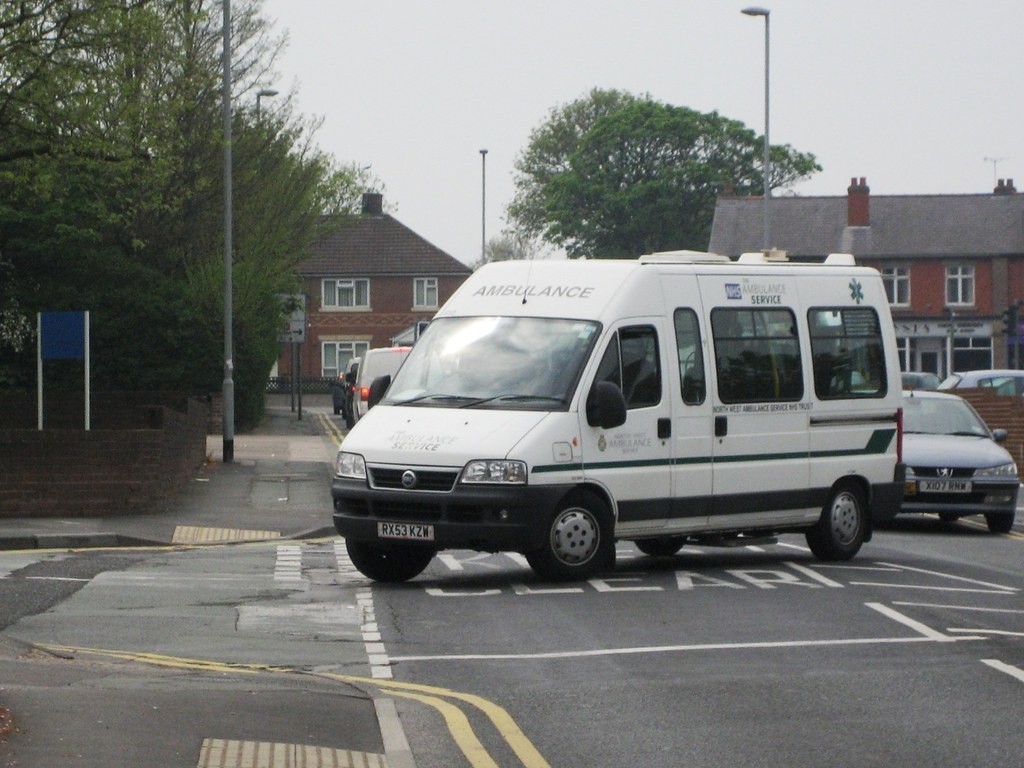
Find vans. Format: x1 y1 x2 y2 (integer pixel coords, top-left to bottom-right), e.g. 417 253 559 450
345 347 412 424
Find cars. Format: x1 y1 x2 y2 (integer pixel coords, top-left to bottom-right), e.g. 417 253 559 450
874 390 1020 533
901 371 943 391
936 369 1024 395
332 357 361 429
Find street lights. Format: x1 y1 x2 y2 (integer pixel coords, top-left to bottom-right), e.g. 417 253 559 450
256 90 279 123
741 7 771 250
480 150 488 260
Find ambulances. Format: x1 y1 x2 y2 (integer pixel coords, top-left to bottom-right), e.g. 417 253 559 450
330 249 905 584
